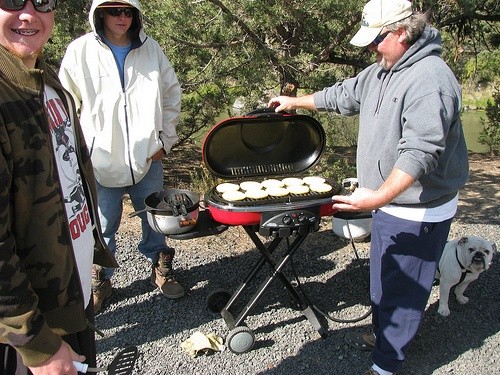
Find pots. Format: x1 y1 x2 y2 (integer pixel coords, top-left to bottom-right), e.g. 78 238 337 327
146 208 199 235
128 188 199 219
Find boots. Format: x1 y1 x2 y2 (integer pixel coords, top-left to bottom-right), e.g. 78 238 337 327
150 248 185 298
92 263 113 313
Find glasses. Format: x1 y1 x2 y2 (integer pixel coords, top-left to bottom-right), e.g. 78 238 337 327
0 0 58 13
368 31 390 49
103 7 135 17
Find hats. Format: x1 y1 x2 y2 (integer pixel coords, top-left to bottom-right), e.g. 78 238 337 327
97 1 133 8
350 0 414 47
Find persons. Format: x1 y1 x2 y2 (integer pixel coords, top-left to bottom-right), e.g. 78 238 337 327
58 0 186 315
0 0 120 375
268 1 468 375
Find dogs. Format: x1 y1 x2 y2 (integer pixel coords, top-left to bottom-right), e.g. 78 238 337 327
434 236 498 317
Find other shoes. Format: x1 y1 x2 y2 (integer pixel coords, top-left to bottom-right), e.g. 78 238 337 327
361 367 397 375
344 332 378 351
